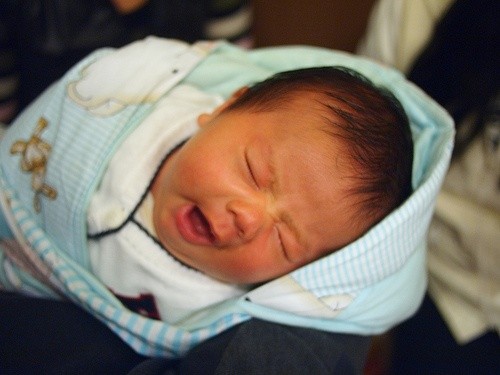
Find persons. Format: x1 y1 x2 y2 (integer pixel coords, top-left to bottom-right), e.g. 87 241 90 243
1 0 253 129
0 34 457 361
1 0 500 374
